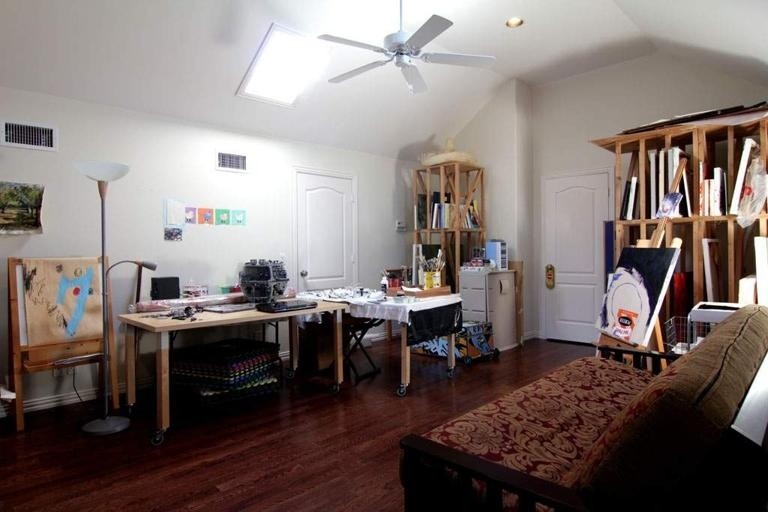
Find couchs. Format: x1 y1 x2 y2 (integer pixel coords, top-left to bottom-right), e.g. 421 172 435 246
399 304 768 512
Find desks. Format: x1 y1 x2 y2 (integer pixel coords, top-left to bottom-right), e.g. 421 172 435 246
291 286 462 396
116 298 350 446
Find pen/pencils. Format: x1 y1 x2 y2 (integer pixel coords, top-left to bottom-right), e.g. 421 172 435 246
416 248 445 271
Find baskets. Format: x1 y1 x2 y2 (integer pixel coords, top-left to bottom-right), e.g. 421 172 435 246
664 316 719 354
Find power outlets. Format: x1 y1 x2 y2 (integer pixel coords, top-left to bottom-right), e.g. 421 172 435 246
67 366 75 375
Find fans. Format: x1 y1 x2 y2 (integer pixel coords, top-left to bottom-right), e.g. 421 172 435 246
317 0 496 94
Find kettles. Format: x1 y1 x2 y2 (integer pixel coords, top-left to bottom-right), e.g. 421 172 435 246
387 275 400 295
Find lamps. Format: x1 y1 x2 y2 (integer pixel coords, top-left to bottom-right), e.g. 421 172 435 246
235 22 346 110
80 160 131 436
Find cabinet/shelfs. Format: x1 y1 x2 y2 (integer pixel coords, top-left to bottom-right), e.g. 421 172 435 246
459 266 519 352
413 159 486 293
591 102 768 375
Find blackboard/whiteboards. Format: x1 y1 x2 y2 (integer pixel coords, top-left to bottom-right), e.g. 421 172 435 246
8 256 114 352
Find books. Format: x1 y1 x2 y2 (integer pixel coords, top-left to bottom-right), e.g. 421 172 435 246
431 191 481 229
